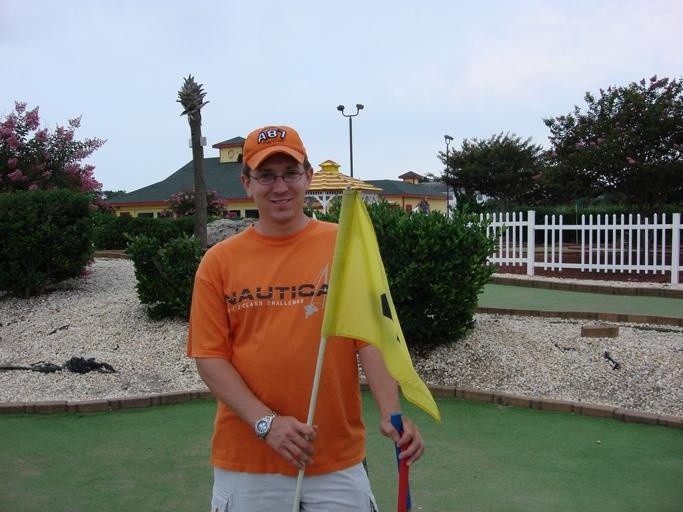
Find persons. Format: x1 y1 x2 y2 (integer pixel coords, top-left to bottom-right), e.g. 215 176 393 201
186 125 425 512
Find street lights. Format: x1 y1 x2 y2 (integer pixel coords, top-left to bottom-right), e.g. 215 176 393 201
334 103 365 181
442 134 455 220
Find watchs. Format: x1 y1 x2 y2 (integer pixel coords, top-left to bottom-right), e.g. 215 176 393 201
254 412 280 440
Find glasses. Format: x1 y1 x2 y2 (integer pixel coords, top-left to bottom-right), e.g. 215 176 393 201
243 169 307 186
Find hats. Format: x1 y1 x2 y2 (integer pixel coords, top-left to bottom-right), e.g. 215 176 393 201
240 124 308 171
